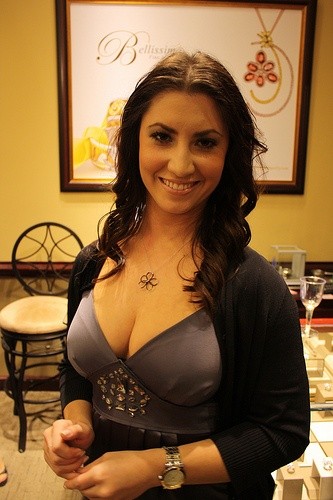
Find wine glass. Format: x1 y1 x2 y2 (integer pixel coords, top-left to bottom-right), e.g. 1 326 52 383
299 276 327 340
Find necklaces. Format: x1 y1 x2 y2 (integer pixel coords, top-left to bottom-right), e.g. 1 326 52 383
132 217 198 292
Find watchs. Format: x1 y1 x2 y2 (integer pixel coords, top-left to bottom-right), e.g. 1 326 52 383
158 445 187 491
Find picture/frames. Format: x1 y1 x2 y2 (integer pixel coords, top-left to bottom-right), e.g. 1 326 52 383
54 0 317 195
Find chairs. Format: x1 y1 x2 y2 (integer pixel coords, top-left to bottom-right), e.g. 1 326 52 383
0 223 83 453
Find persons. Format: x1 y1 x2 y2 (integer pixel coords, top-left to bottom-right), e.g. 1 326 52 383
42 51 306 500
